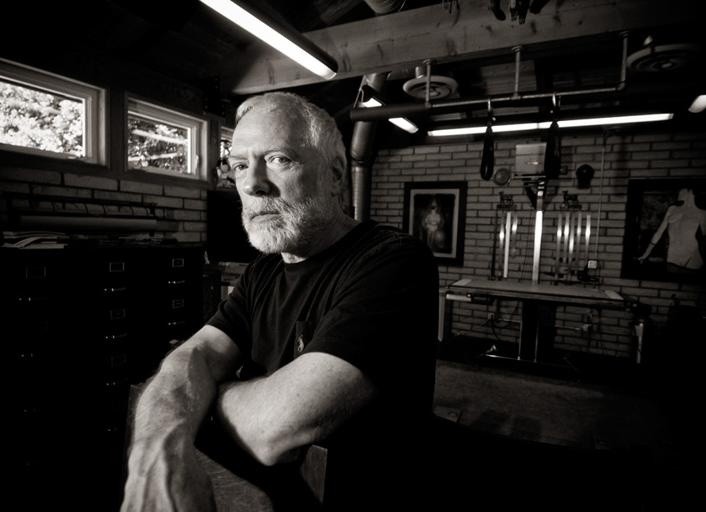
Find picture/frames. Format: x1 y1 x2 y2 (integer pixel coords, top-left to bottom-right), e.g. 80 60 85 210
403 180 467 267
620 179 706 286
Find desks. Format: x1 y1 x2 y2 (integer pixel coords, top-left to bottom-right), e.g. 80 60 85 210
449 279 626 365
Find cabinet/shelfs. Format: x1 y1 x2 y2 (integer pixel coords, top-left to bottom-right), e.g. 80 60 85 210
2 242 205 462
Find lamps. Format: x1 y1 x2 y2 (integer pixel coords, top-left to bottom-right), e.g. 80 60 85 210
403 75 458 99
202 1 339 82
627 42 692 74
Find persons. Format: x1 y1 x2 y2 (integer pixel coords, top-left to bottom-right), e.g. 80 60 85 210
638 188 704 270
117 90 442 512
424 200 440 247
